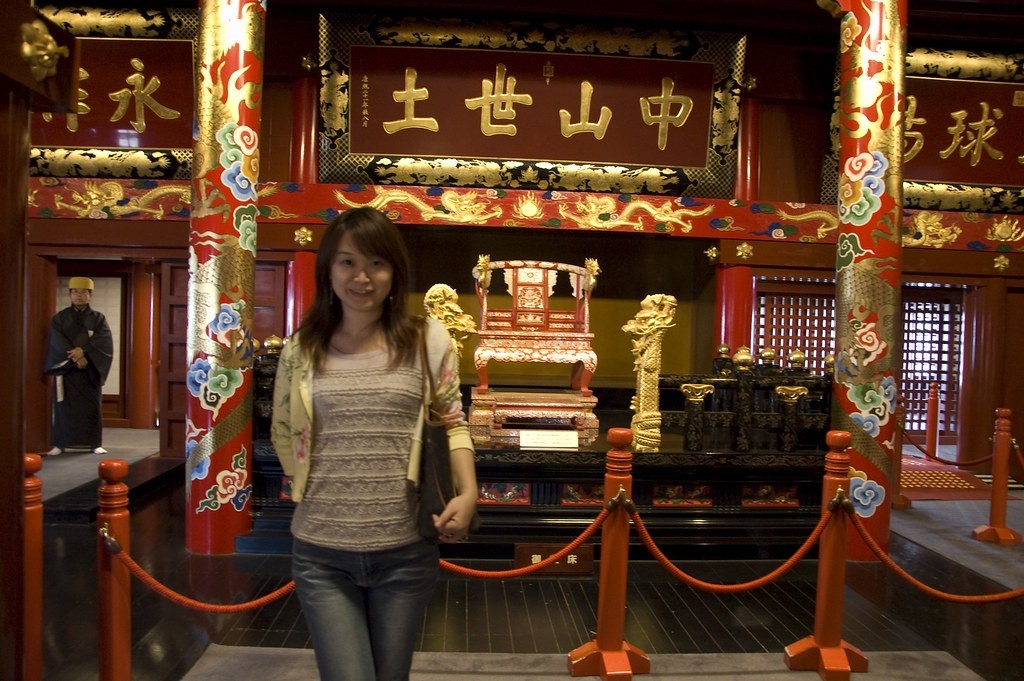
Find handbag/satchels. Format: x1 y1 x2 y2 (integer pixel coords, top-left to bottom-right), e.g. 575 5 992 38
416 315 481 540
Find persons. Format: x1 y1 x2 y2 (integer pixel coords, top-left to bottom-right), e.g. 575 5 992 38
271 206 478 681
43 276 114 455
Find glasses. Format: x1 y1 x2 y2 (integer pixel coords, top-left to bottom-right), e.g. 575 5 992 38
71 291 89 295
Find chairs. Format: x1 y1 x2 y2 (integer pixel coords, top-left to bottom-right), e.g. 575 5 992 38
470 253 603 429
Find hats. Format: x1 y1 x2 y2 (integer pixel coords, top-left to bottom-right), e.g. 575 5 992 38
69 277 94 291
